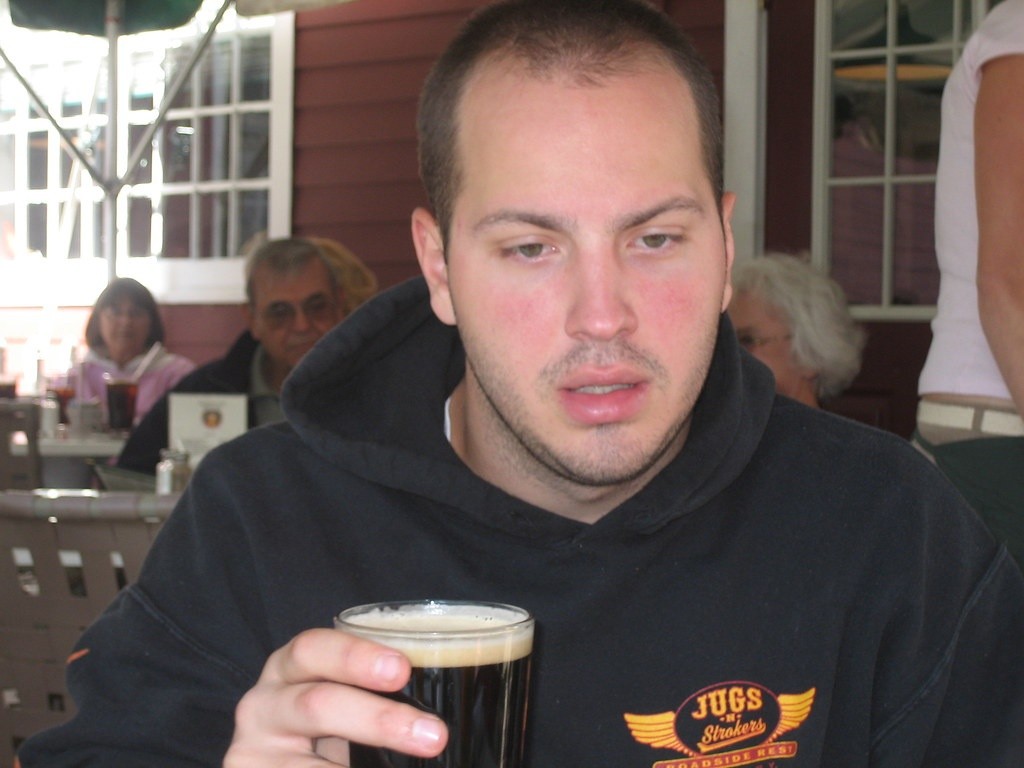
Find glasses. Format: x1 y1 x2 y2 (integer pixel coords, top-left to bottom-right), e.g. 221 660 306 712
736 328 793 347
265 293 329 327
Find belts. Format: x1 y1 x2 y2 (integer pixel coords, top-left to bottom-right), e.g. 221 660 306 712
916 400 1024 437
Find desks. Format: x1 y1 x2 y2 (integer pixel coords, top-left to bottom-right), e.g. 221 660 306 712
11 425 131 487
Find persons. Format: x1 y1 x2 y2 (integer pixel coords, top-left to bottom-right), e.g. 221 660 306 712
18 0 1024 768
892 0 1024 568
40 278 195 491
118 236 378 479
728 254 866 411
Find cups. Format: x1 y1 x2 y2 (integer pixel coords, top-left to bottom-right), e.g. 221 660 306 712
45 374 77 431
101 372 139 438
333 597 534 768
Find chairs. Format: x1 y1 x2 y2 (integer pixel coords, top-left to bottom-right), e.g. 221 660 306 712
0 397 45 489
0 488 182 768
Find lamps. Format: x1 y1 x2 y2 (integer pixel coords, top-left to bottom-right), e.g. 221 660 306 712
833 0 953 84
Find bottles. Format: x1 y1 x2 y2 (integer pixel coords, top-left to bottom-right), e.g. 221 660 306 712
155 448 175 493
170 453 191 492
41 392 59 433
